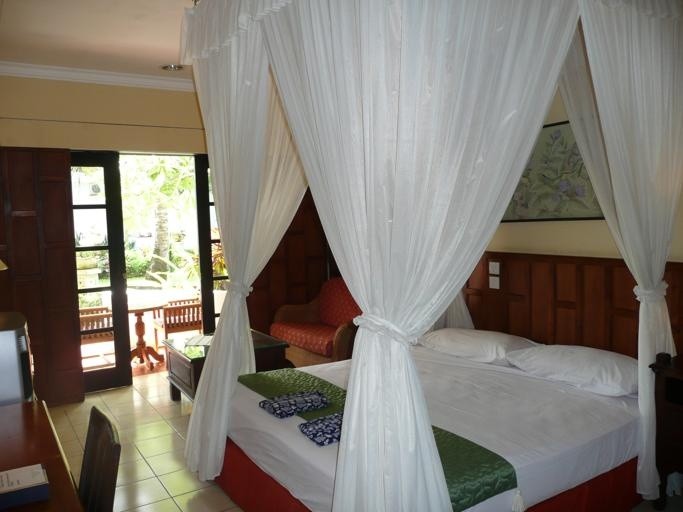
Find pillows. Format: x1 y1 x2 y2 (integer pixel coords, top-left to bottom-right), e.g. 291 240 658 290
417 326 637 401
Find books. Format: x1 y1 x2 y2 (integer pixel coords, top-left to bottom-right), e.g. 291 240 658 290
0 463 50 494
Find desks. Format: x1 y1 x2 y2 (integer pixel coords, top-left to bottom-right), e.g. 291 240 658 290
127 303 164 370
1 399 89 511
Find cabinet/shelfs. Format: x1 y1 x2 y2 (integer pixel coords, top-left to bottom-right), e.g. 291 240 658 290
647 355 683 512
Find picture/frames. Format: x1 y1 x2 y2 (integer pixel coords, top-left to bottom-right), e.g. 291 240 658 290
498 119 609 223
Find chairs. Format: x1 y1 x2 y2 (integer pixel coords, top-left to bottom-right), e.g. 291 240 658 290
79 305 116 370
76 405 122 512
269 277 361 367
150 299 203 371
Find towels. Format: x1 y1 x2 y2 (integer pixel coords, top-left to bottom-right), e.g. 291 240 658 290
258 389 342 448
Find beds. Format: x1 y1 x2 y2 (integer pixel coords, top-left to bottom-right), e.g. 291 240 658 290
207 248 682 512
